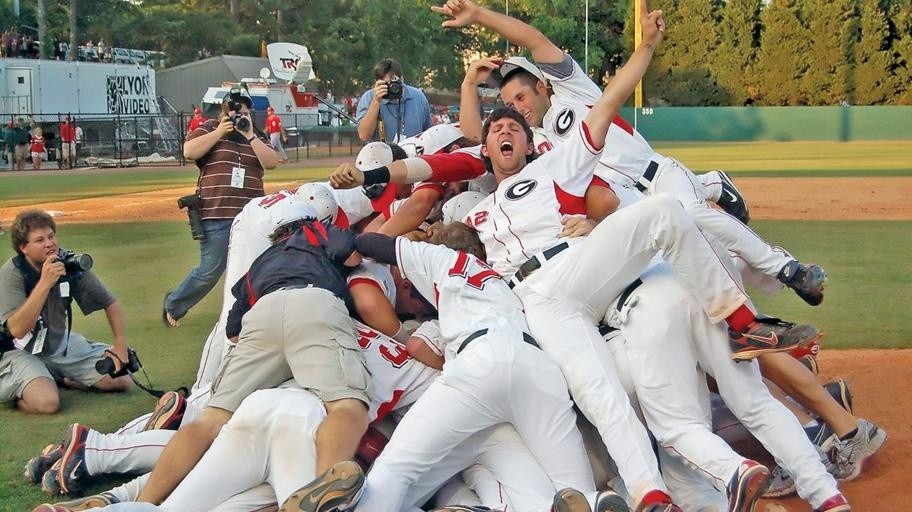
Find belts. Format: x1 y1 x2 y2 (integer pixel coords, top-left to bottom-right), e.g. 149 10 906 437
598 326 620 337
258 280 347 304
506 241 569 291
615 278 644 312
455 328 544 355
635 158 659 193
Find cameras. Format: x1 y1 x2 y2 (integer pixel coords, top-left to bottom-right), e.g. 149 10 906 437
96 348 140 379
177 193 207 240
227 87 250 132
52 249 93 283
381 81 404 100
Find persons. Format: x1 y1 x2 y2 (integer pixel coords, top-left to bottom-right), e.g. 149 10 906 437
13 117 32 171
1 22 17 58
0 207 135 416
2 121 16 171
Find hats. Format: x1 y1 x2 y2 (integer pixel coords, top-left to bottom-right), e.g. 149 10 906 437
223 87 254 110
397 136 421 159
295 182 339 230
266 106 274 113
270 194 320 233
354 140 394 199
415 122 466 160
194 107 202 113
486 55 547 90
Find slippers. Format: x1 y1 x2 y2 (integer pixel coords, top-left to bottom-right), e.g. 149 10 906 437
162 291 187 328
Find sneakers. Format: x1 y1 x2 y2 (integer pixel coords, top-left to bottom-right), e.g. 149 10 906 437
55 422 88 499
726 313 825 364
799 354 820 377
830 417 888 483
549 485 593 512
30 492 121 512
275 460 368 511
801 420 837 453
22 443 64 498
822 377 853 419
593 486 630 511
760 445 836 500
278 159 288 165
138 389 189 432
778 260 828 307
715 168 749 227
724 457 772 512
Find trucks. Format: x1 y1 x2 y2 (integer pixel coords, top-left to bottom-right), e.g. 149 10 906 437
195 78 321 151
2 56 169 164
